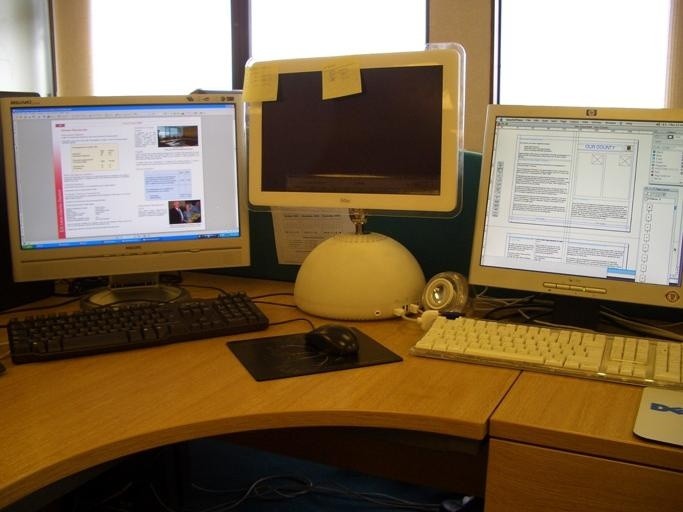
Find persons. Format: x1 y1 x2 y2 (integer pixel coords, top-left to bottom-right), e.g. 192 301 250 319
169 201 190 223
183 202 196 224
192 200 200 214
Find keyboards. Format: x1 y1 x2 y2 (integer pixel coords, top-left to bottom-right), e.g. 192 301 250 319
408 315 683 391
6 290 269 365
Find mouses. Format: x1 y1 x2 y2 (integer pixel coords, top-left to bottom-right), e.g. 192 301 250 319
304 324 359 358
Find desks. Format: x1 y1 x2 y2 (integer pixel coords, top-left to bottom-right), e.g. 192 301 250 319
1 274 683 511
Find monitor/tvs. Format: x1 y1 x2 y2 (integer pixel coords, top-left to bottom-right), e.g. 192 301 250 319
466 104 683 331
248 50 460 212
0 94 252 310
